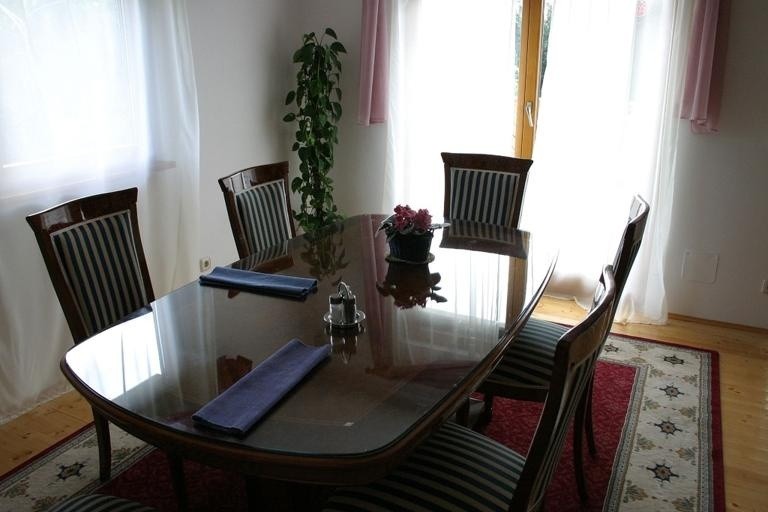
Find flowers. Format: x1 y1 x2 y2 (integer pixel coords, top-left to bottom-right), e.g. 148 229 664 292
374 202 454 233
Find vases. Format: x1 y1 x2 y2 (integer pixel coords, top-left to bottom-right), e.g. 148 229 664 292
383 225 434 262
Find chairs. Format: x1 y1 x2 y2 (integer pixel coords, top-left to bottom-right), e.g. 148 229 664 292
324 259 617 512
25 186 193 508
476 206 651 512
440 152 532 229
218 162 297 262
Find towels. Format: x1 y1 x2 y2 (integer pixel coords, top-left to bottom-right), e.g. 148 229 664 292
190 328 334 440
194 265 321 302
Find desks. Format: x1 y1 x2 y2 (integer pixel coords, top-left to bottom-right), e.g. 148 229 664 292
53 214 554 509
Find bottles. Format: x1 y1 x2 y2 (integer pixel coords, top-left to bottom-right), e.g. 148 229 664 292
329 335 343 357
341 335 357 364
328 294 344 321
342 292 357 322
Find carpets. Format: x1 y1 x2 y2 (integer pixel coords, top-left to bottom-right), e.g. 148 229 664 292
1 330 727 512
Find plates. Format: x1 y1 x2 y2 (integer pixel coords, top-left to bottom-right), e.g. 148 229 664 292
325 327 366 337
323 309 367 326
